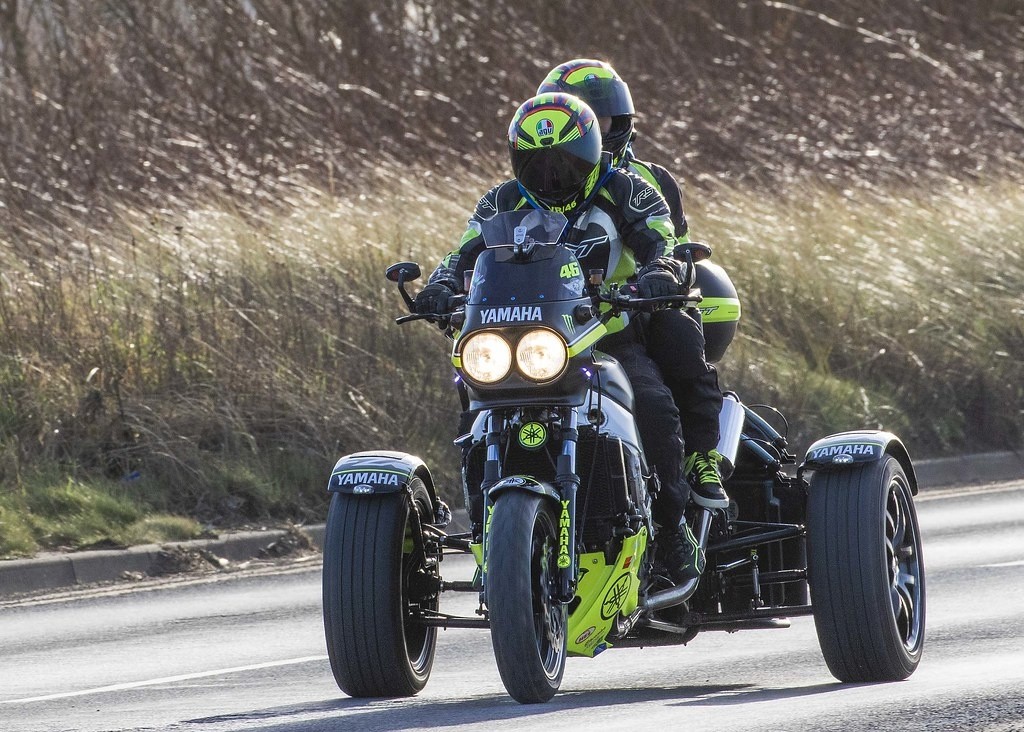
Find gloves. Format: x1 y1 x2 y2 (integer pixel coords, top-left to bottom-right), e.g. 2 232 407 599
414 285 455 330
637 265 679 313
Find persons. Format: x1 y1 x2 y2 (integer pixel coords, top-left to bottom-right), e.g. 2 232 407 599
414 91 707 581
537 60 729 509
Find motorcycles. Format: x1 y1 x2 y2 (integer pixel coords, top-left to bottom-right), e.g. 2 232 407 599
321 208 928 704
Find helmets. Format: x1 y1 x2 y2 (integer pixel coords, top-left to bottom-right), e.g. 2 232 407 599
507 93 603 217
537 58 634 164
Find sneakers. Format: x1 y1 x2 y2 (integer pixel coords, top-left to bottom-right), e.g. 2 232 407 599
656 522 706 584
683 448 729 509
